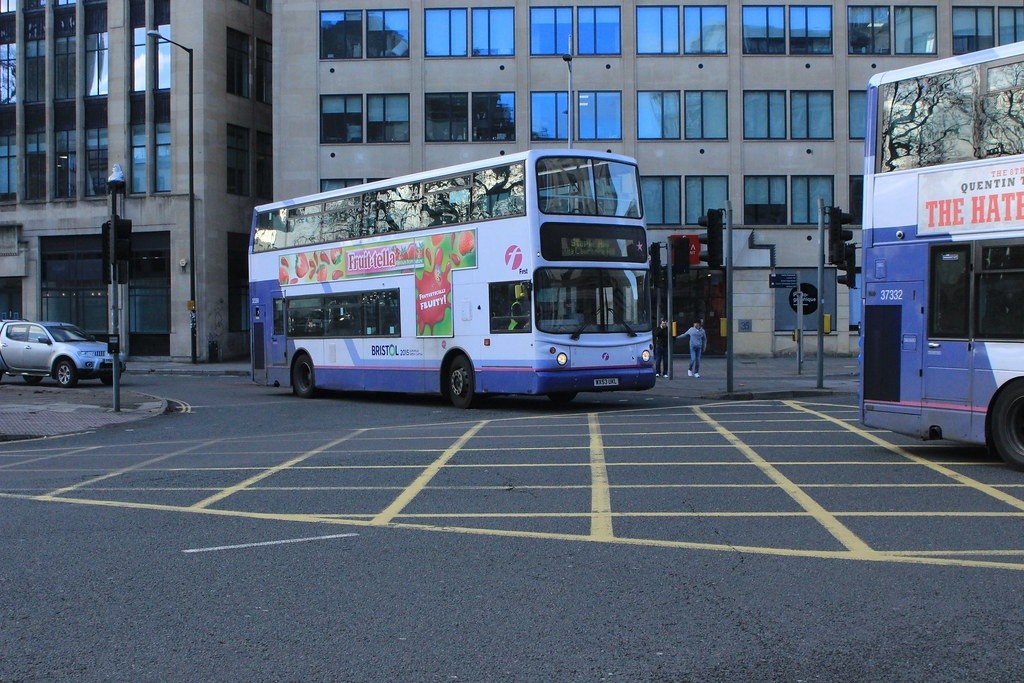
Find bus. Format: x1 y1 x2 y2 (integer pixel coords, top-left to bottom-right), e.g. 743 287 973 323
246 149 659 409
855 40 1024 473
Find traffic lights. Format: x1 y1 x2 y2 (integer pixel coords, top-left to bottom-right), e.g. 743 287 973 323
835 243 857 289
108 213 133 264
697 208 724 268
827 207 854 266
673 235 690 275
823 313 832 335
647 242 661 289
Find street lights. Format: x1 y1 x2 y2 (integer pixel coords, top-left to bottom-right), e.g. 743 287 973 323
562 34 575 150
146 29 197 363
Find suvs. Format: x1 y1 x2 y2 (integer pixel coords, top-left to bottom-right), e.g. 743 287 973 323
0 317 128 388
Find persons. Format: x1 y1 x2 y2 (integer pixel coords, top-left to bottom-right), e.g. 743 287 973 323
676 319 707 378
654 318 676 378
507 292 528 331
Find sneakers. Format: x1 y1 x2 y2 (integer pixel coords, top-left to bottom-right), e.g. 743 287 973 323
688 370 693 376
695 373 700 377
663 375 668 378
656 374 659 376
